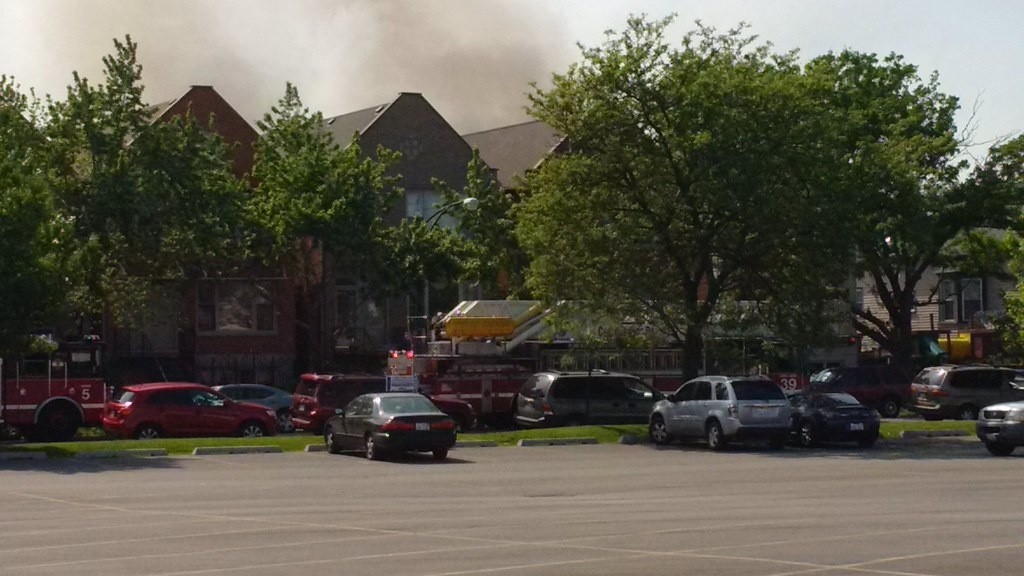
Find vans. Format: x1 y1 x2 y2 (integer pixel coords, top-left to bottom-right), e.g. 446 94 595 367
909 364 1024 420
290 371 386 436
802 365 910 419
510 368 666 428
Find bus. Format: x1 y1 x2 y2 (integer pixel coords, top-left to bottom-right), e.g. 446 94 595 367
423 354 538 434
0 333 108 442
539 342 810 399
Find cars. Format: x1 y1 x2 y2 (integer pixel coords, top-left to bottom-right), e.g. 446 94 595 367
98 382 278 439
322 392 458 460
210 384 296 434
975 400 1024 455
788 386 881 449
647 373 793 450
